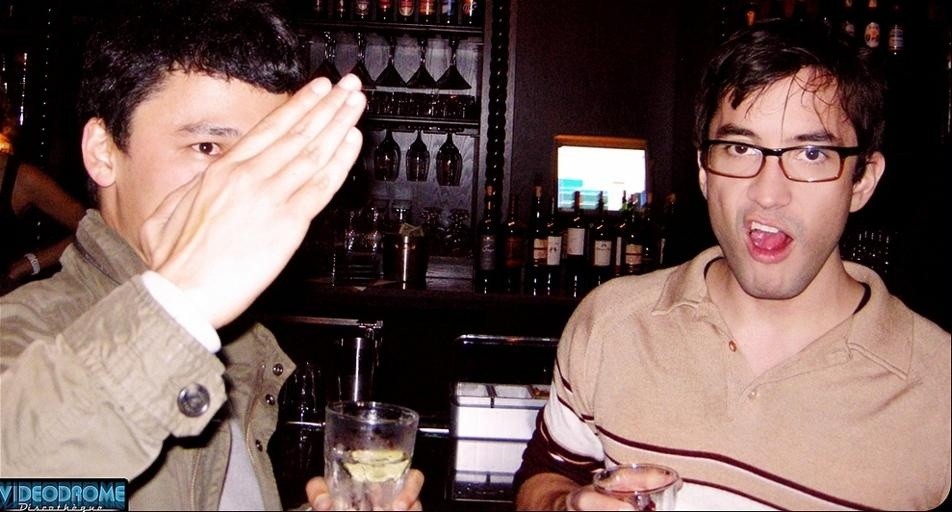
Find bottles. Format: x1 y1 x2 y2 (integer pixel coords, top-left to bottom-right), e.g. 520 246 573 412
312 0 482 27
738 1 906 56
341 198 467 258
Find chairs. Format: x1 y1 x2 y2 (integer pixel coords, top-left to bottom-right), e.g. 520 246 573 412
450 328 560 384
271 311 388 463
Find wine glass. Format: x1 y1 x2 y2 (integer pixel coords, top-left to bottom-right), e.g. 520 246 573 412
375 125 463 187
473 184 683 291
306 27 475 124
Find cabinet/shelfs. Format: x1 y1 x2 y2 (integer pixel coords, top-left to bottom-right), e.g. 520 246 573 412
303 1 500 289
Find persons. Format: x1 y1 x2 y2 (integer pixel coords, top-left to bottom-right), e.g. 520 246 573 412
0 2 425 512
1 150 84 301
512 19 951 511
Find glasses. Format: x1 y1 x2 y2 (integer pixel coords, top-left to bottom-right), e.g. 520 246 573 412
700 136 862 184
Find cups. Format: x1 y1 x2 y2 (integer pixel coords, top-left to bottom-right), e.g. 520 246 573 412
591 464 681 511
322 397 420 510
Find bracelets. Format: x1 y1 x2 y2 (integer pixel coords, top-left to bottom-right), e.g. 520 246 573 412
24 252 41 276
